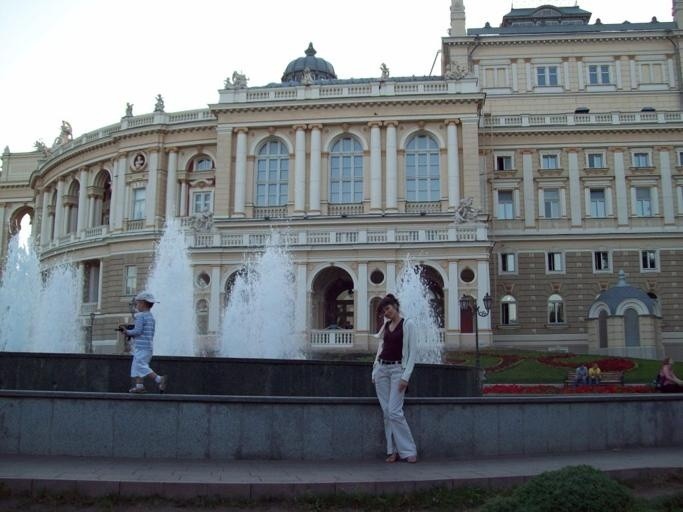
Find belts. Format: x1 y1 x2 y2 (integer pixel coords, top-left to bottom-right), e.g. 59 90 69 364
378 359 401 364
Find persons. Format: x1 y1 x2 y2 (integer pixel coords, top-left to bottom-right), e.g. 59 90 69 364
369 293 417 464
585 362 603 387
570 362 587 389
119 290 169 396
320 306 353 344
656 356 682 387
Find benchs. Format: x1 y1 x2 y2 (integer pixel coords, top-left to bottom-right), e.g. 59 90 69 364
653 373 678 393
563 370 625 388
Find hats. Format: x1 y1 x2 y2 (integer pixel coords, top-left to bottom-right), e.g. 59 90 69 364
128 387 146 393
135 291 160 303
157 374 169 394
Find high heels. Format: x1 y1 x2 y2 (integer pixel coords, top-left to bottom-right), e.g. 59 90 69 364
386 452 417 463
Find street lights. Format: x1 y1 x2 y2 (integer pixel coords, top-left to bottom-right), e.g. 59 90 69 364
460 293 492 369
